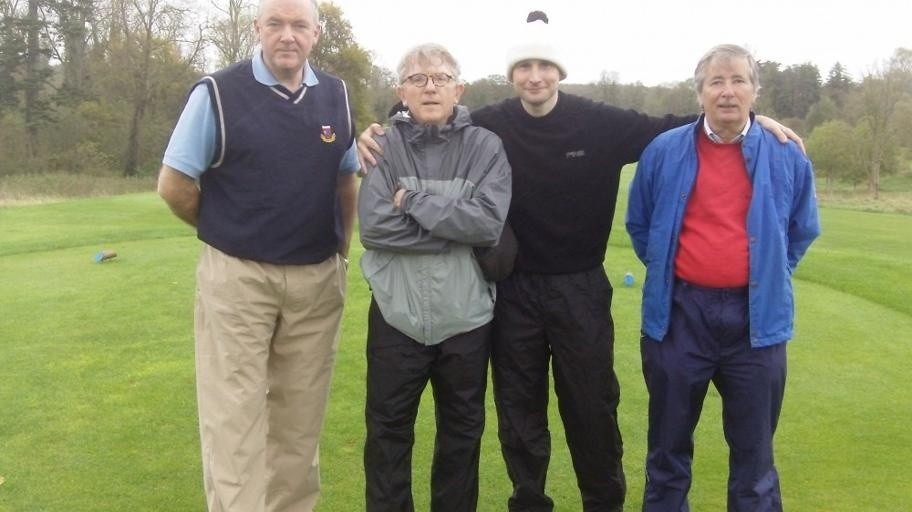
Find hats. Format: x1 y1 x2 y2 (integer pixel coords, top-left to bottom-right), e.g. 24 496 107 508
507 20 569 82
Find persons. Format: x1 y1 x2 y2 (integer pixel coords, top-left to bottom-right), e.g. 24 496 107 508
626 44 820 512
158 0 361 512
356 12 806 512
357 43 514 512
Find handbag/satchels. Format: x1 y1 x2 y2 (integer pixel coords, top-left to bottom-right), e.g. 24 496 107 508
473 217 519 282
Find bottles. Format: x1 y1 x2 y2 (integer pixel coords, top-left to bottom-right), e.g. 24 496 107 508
623 273 634 286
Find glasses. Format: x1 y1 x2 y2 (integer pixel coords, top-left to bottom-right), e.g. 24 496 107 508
401 72 457 88
